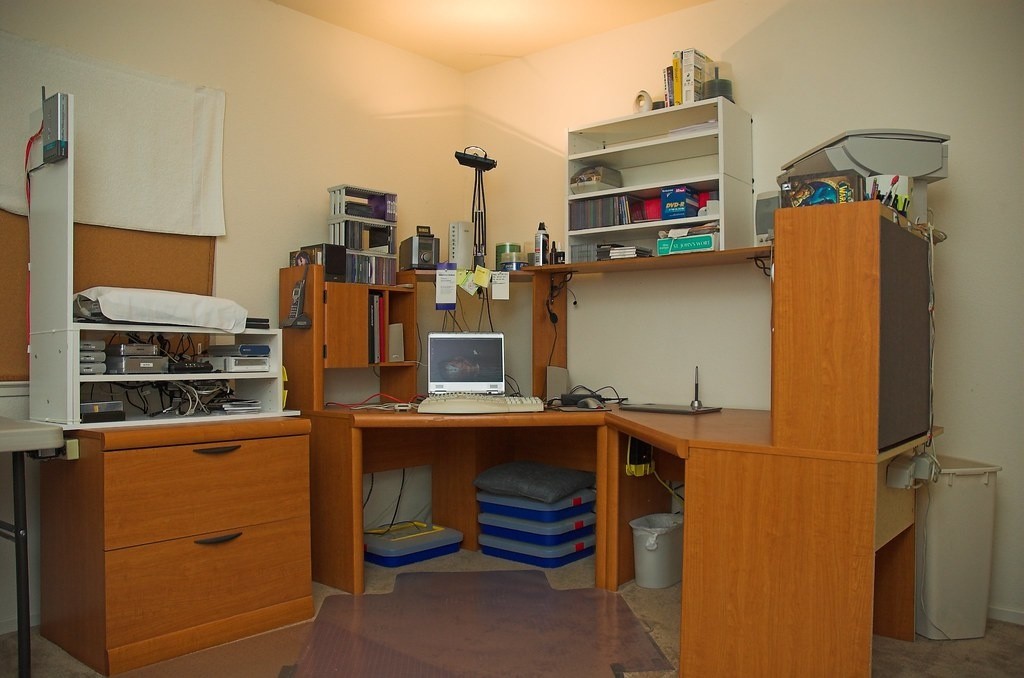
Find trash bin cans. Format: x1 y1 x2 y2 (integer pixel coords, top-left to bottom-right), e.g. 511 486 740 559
914 454 1003 640
629 513 684 589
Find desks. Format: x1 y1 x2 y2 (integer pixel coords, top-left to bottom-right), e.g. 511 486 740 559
305 401 946 678
0 417 66 678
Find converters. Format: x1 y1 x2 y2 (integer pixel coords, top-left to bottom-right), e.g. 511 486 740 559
561 393 603 406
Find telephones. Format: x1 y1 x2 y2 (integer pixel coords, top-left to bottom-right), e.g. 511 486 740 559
279 265 311 328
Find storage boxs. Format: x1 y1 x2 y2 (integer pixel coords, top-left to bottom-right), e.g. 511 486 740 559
365 489 597 568
656 232 720 256
660 185 700 221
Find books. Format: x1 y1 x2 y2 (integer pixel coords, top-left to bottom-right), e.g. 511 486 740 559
660 48 714 108
369 294 385 364
208 399 261 415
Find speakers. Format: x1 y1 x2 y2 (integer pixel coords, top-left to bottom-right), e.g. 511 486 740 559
547 366 568 406
388 322 404 362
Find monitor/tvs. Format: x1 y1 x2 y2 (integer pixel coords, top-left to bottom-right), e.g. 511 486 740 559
426 330 506 396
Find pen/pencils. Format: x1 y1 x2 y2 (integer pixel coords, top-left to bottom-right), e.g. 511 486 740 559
864 175 911 219
695 366 698 401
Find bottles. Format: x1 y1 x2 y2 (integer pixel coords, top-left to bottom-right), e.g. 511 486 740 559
495 242 521 272
550 241 565 265
535 222 550 266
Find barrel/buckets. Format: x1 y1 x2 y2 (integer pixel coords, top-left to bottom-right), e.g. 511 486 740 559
913 454 1003 640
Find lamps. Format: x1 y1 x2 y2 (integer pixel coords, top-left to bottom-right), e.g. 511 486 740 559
454 144 498 270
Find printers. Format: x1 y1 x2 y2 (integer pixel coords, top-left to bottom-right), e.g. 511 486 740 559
777 127 951 224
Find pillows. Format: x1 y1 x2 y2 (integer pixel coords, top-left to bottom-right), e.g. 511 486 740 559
474 462 594 503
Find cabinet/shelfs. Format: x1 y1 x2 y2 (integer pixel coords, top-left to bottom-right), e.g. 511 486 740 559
279 264 418 410
770 199 935 450
565 95 753 263
70 324 282 426
332 184 401 274
44 416 311 678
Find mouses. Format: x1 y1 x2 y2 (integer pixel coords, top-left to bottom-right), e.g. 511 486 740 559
577 398 604 409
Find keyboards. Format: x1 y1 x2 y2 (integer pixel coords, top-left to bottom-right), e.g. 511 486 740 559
418 395 544 414
